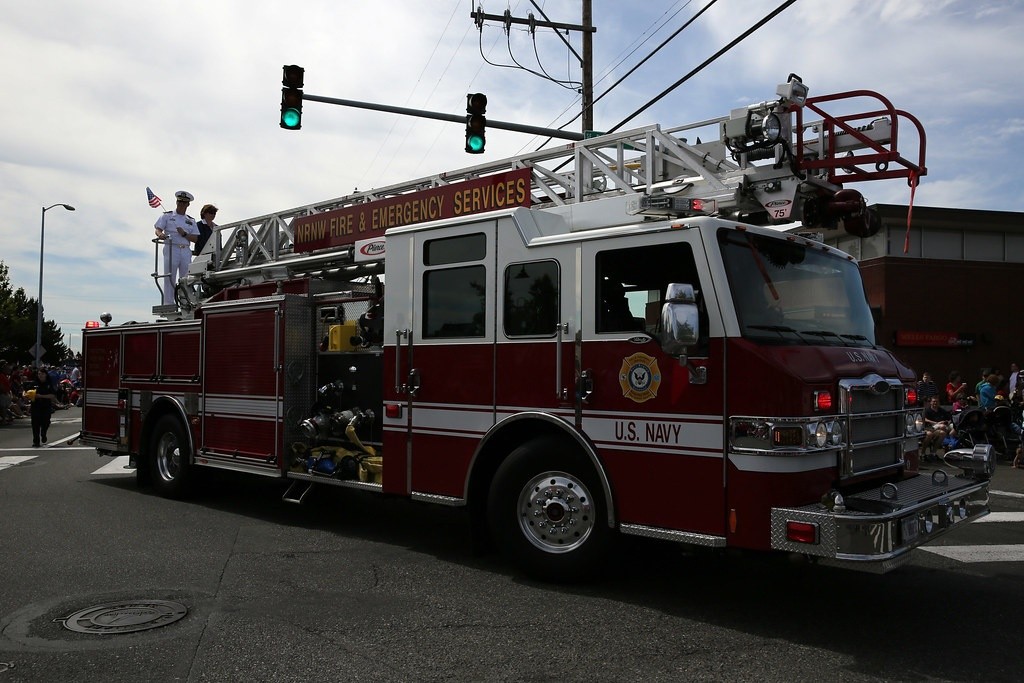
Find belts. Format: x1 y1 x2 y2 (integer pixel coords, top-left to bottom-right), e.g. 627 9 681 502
165 244 189 248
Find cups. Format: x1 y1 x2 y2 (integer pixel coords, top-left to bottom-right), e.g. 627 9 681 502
962 383 966 388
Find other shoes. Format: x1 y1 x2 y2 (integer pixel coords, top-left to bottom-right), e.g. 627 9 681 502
32 443 40 447
41 437 47 443
920 454 928 463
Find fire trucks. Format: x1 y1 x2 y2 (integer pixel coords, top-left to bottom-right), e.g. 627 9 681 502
66 73 996 575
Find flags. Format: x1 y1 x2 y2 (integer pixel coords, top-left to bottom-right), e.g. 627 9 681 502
146 186 161 208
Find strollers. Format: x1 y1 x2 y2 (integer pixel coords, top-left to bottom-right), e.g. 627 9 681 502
984 406 1022 461
949 409 1003 459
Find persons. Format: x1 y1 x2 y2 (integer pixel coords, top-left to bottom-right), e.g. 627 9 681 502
154 191 201 305
0 358 85 428
194 204 222 258
916 360 1024 470
26 367 57 448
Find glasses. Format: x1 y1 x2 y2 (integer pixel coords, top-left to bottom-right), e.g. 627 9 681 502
207 210 216 214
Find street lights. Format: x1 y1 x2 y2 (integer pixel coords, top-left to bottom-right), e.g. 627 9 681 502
36 204 75 368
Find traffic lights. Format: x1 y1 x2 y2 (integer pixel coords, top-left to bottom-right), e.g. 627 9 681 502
280 65 305 130
465 93 487 154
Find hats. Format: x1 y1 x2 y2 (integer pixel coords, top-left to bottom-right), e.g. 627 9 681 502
175 191 194 202
201 205 218 219
1018 370 1024 378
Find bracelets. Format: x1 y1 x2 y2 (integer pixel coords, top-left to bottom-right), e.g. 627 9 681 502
182 232 187 237
